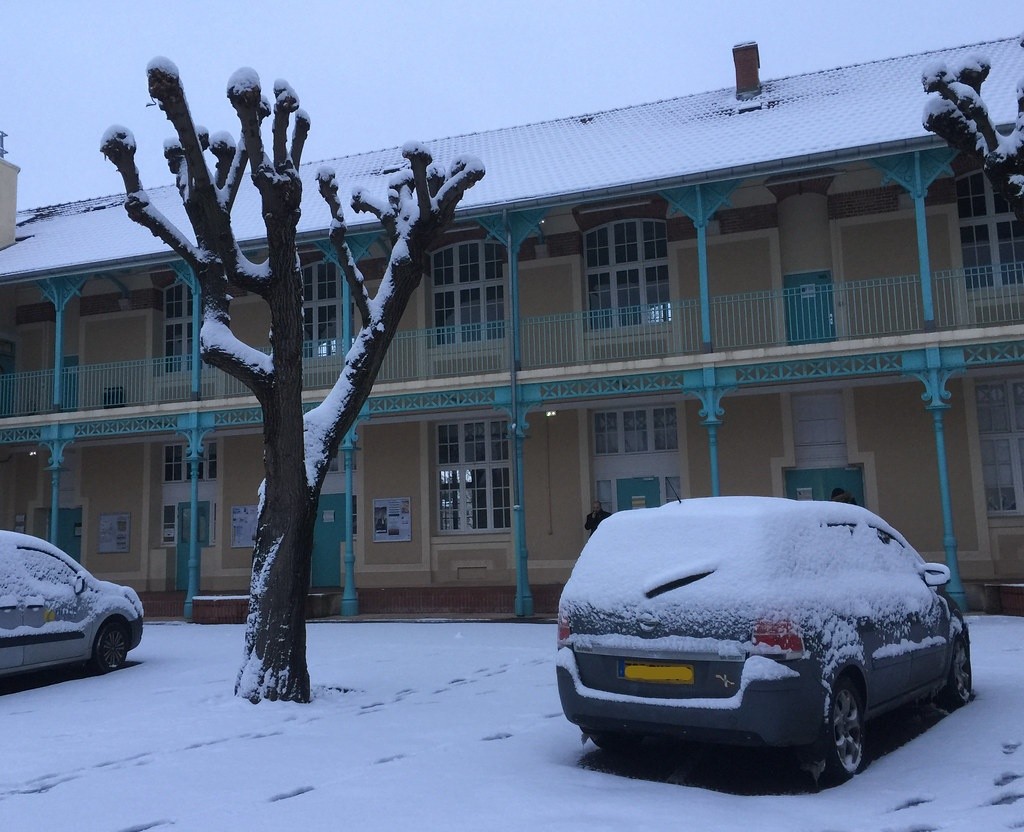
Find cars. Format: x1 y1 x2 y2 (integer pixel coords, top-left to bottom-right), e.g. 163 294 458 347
553 490 971 785
0 527 145 694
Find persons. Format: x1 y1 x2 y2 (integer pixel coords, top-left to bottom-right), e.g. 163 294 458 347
831 488 856 504
401 501 409 513
585 501 612 537
376 508 387 530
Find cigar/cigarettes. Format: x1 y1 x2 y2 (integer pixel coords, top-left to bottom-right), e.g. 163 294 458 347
594 511 596 513
